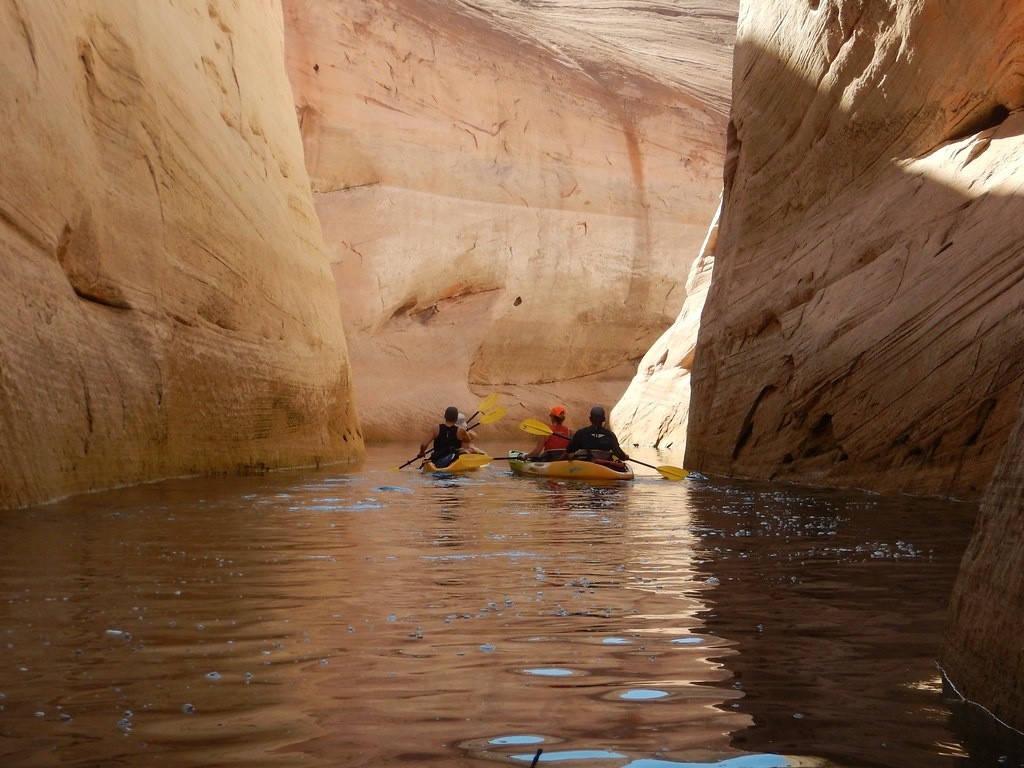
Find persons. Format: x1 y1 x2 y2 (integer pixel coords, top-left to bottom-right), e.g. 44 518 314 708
457 413 476 449
416 406 470 469
567 407 629 463
521 406 573 462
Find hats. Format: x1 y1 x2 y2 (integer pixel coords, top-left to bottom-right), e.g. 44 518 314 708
590 407 605 419
454 413 467 431
549 405 567 418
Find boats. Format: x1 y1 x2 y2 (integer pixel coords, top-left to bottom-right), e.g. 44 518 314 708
419 445 491 476
507 448 638 479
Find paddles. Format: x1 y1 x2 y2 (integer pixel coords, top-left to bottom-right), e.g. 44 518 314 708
522 419 689 482
418 391 500 470
392 406 507 472
457 452 539 468
